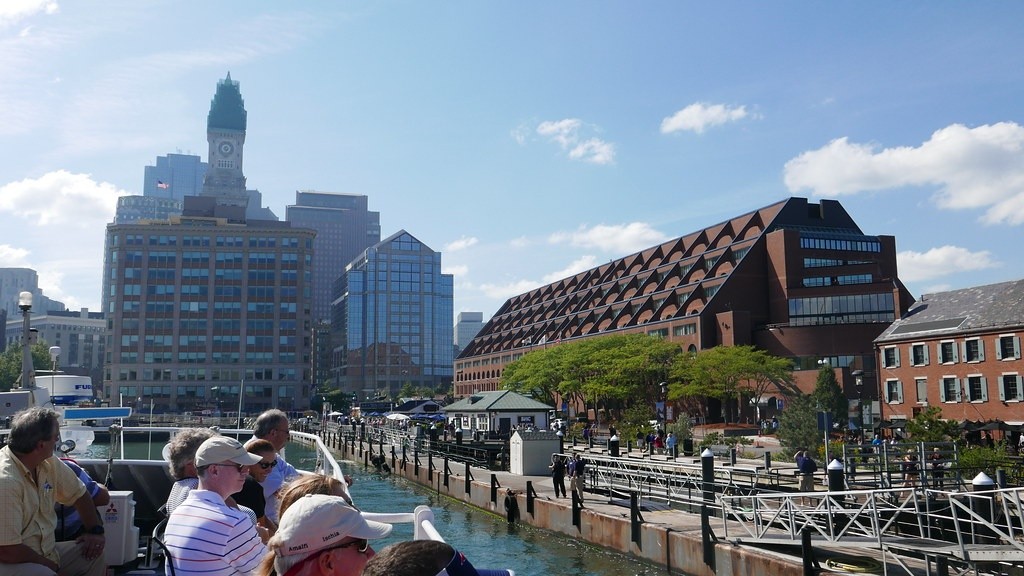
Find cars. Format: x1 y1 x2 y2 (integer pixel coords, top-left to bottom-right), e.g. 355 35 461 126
569 417 588 425
550 418 567 429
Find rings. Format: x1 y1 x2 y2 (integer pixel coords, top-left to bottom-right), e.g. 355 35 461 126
100 546 104 549
95 544 100 547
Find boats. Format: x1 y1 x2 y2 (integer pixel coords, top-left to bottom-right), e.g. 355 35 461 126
0 291 133 456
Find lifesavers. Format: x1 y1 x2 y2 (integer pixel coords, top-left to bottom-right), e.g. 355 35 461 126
504 495 517 513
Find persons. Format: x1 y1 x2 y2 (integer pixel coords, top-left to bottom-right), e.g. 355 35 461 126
609 424 617 438
636 425 676 456
794 451 817 507
0 407 110 576
551 419 567 438
496 424 538 440
900 449 917 498
549 452 584 502
855 433 902 459
760 419 778 431
964 430 994 448
1020 433 1024 446
928 448 945 490
582 421 597 446
164 410 514 576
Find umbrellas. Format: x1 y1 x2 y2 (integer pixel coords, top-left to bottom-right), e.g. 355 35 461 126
956 419 1024 439
386 414 409 420
872 420 908 429
328 411 343 416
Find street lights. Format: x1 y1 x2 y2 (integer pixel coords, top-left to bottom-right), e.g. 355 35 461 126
18 291 38 390
851 369 865 445
660 382 671 436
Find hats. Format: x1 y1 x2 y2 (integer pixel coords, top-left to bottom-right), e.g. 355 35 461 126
268 494 393 557
435 549 515 576
194 435 263 469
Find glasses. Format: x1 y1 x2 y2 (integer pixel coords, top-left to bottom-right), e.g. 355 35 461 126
268 429 289 436
258 461 277 468
344 498 352 506
203 463 244 471
42 432 60 441
305 538 368 561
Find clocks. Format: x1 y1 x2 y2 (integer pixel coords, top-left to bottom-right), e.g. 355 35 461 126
219 141 235 157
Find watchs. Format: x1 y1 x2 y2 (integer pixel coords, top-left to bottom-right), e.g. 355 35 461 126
87 525 105 534
